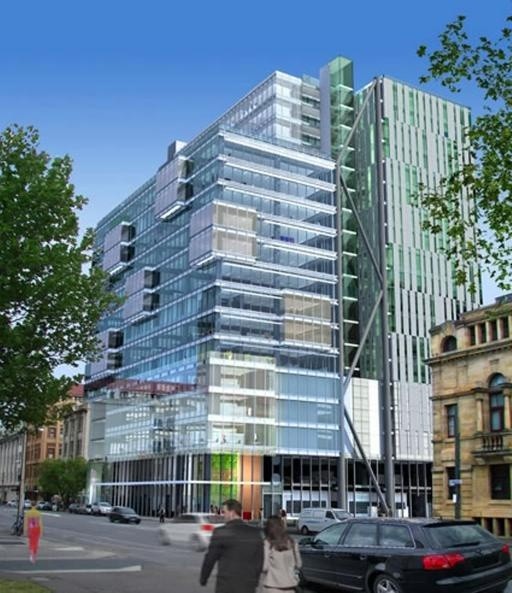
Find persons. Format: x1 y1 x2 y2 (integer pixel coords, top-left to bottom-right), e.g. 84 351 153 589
200 500 264 593
156 503 287 523
24 499 44 564
257 515 303 593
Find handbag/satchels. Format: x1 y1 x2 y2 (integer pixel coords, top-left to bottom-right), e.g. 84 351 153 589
294 566 305 592
